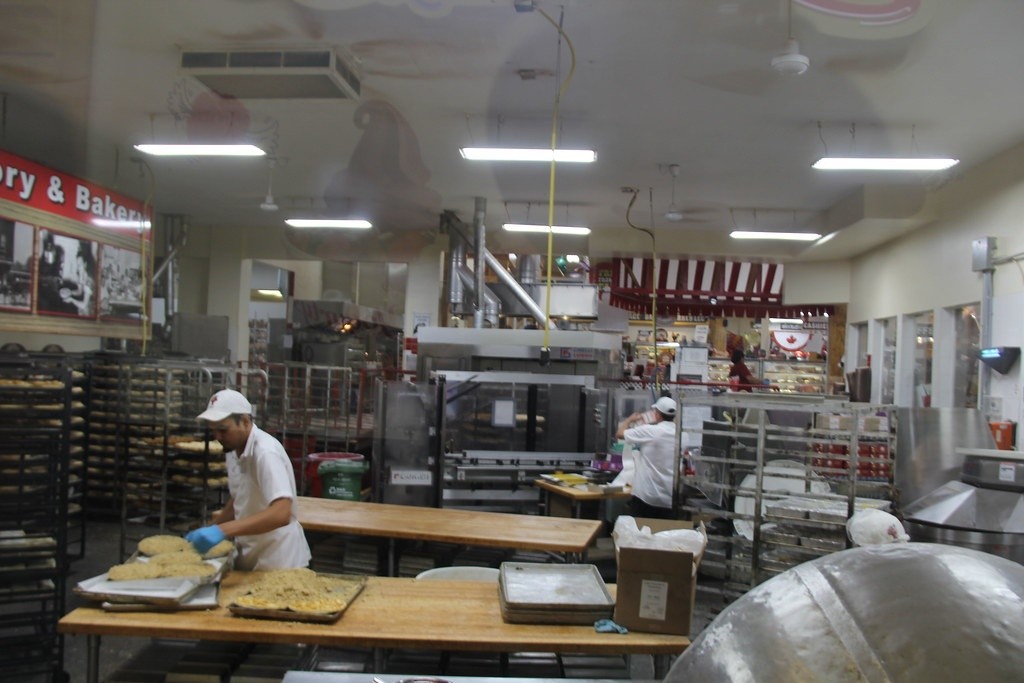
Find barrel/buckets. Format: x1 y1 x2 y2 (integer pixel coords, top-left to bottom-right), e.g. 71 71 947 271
308 452 364 498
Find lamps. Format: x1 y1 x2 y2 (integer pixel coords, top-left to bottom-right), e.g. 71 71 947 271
729 207 822 243
458 112 599 163
132 112 268 157
501 200 593 236
811 121 961 172
284 196 373 229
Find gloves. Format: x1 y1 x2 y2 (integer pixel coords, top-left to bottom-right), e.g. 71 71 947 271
185 524 225 554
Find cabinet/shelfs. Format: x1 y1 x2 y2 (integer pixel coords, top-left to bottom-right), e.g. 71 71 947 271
707 356 830 397
0 297 420 683
669 392 904 624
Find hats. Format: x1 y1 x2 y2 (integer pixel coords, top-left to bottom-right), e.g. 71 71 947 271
194 388 252 423
651 397 677 415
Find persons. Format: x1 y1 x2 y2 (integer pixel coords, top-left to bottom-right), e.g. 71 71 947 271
616 396 685 518
35 234 97 319
184 389 313 573
729 349 780 394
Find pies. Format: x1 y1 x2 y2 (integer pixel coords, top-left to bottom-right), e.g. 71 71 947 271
233 567 347 615
107 534 234 581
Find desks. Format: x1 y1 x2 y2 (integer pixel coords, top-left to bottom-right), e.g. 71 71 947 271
210 494 605 578
57 569 692 683
534 478 633 564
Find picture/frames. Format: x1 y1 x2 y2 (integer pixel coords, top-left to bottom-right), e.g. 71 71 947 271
35 225 101 320
0 214 37 314
97 240 150 326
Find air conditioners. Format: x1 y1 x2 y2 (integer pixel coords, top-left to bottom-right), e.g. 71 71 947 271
178 40 364 101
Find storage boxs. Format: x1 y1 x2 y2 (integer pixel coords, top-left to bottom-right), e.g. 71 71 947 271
610 514 707 636
